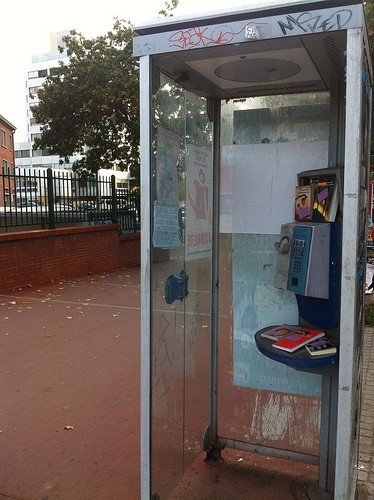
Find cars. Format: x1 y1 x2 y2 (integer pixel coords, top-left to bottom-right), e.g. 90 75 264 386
98 198 128 210
16 200 96 211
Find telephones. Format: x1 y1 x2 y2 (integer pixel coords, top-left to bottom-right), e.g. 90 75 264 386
275 223 329 298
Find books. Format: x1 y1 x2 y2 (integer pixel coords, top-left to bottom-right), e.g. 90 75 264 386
312 177 339 222
272 327 325 353
260 323 295 341
294 185 314 221
305 335 337 356
